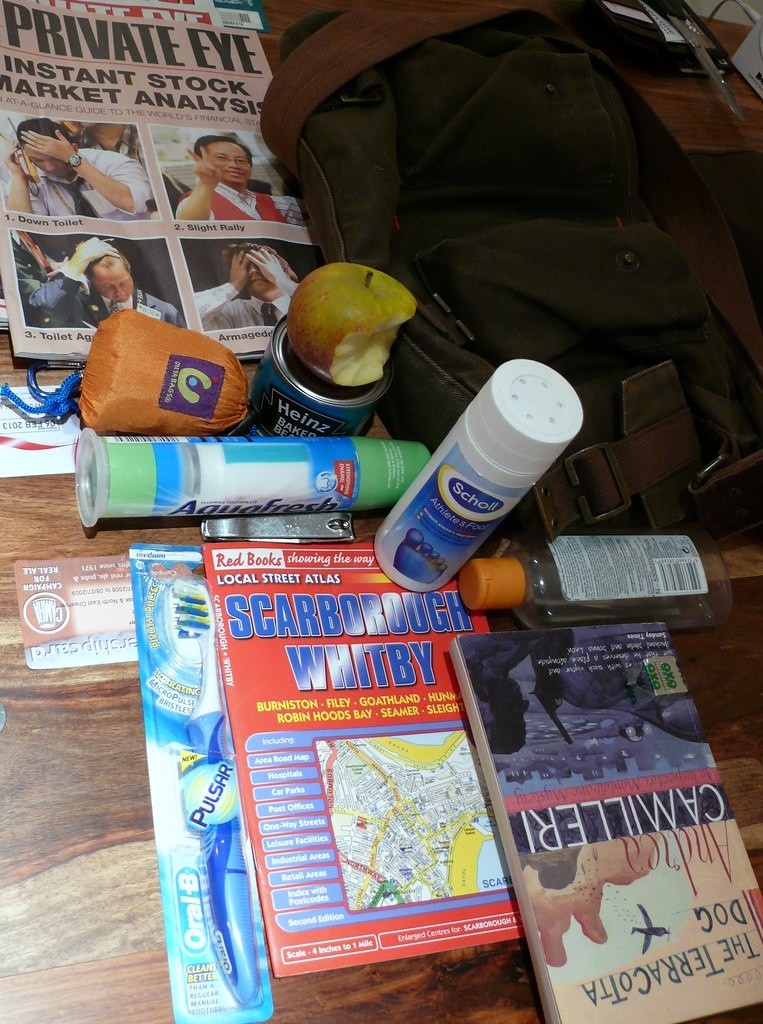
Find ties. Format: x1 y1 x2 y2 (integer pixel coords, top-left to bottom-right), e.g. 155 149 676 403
259 303 278 326
55 177 99 218
109 300 118 314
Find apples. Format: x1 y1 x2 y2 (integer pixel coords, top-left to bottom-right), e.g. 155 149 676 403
289 262 416 385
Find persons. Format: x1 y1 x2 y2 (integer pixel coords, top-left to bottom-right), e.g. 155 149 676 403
11 229 90 326
3 118 154 220
175 135 286 222
28 237 187 332
194 243 299 332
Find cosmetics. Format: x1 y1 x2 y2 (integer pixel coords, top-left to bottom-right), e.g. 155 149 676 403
459 533 733 629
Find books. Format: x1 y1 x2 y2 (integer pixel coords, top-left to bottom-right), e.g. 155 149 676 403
204 542 526 978
449 621 763 1024
0 0 327 361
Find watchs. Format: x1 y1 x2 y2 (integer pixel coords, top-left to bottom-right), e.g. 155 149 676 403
67 155 82 168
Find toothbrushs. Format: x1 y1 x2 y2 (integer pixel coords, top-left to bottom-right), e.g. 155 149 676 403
167 578 259 1007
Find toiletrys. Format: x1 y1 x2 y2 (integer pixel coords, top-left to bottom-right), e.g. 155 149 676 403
454 528 735 635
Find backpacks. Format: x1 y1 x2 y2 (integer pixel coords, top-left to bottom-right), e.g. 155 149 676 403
256 0 763 541
